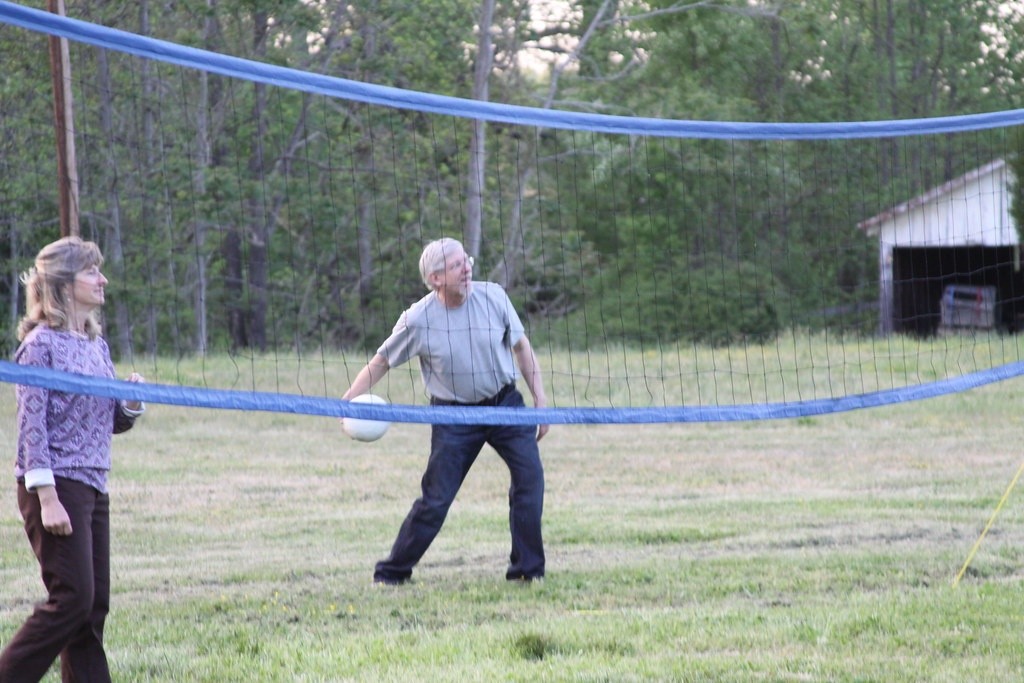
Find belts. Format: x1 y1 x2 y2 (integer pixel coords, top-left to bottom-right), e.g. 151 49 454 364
475 386 511 407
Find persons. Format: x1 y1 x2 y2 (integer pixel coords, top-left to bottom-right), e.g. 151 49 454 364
0 237 146 683
335 238 549 586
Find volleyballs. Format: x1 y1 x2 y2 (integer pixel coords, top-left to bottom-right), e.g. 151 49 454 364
342 393 392 443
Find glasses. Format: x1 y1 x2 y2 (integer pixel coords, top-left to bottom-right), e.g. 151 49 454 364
436 256 474 275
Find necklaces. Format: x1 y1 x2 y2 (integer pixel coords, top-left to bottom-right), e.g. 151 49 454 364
69 328 89 340
